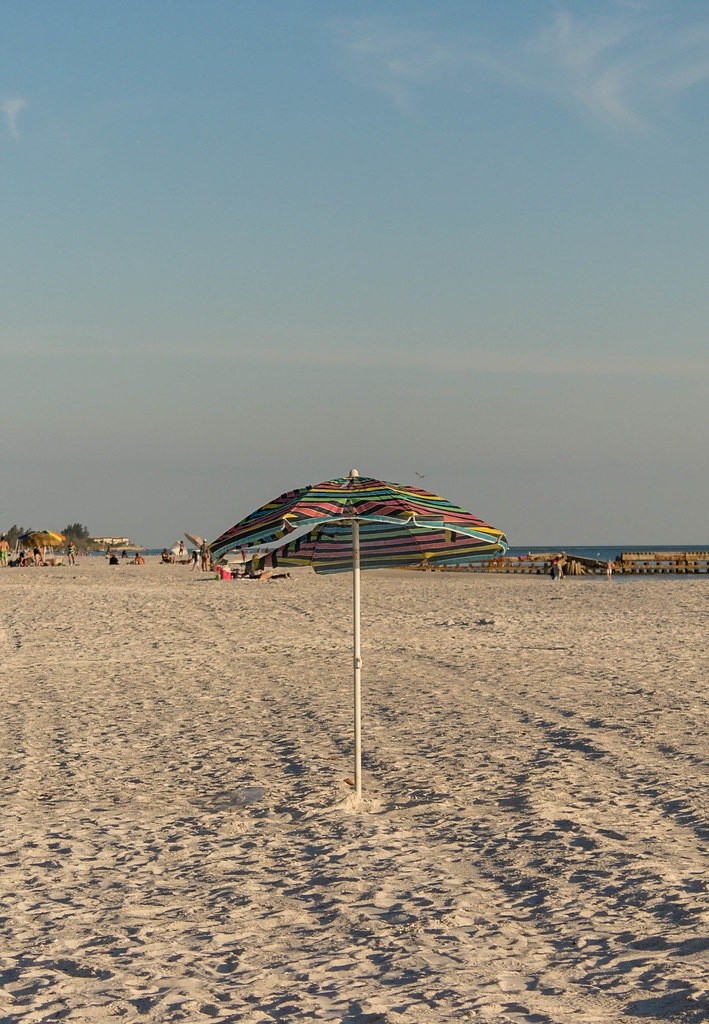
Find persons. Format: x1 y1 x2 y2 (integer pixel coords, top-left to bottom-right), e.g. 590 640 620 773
241 550 292 579
9 546 41 567
604 559 614 578
179 541 185 556
615 556 622 573
67 540 77 565
551 551 568 580
135 553 145 565
162 548 175 564
122 550 127 558
0 536 10 566
191 538 210 571
518 552 534 562
104 546 121 565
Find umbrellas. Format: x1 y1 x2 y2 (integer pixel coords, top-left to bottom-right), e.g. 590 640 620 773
208 468 511 793
18 529 66 561
